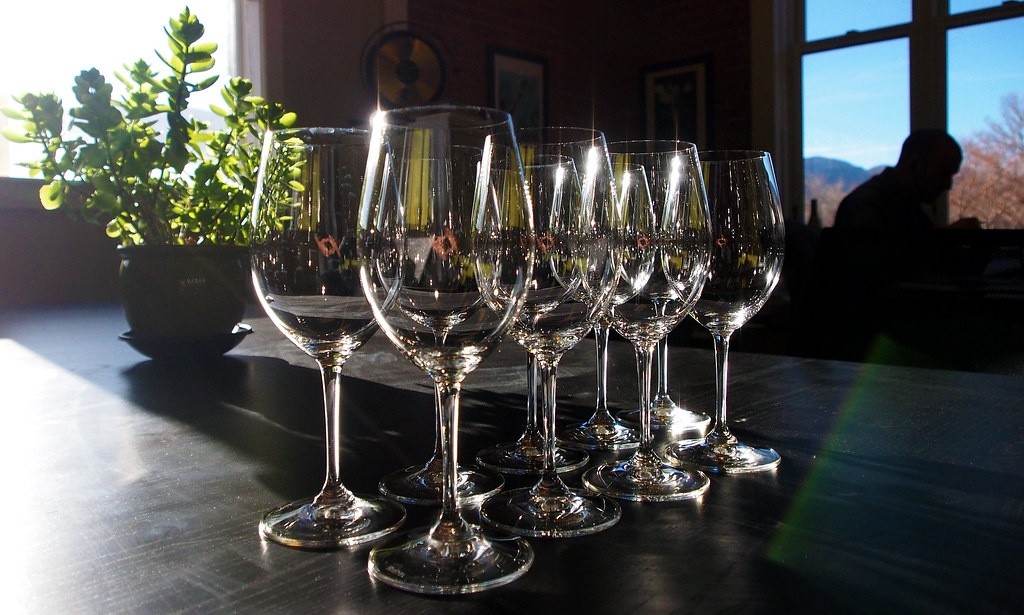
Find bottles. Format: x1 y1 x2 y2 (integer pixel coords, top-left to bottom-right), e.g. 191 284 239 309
807 198 822 235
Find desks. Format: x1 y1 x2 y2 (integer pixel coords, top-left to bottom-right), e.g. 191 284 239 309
0 305 1024 615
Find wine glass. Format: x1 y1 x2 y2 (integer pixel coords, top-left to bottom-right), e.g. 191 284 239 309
247 125 408 549
368 104 787 593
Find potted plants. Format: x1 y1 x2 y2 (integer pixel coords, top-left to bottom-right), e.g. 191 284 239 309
0 6 307 369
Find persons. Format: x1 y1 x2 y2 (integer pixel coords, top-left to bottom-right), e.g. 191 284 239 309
833 127 984 287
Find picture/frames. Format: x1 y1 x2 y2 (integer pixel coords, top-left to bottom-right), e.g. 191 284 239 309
484 45 548 155
638 52 711 169
